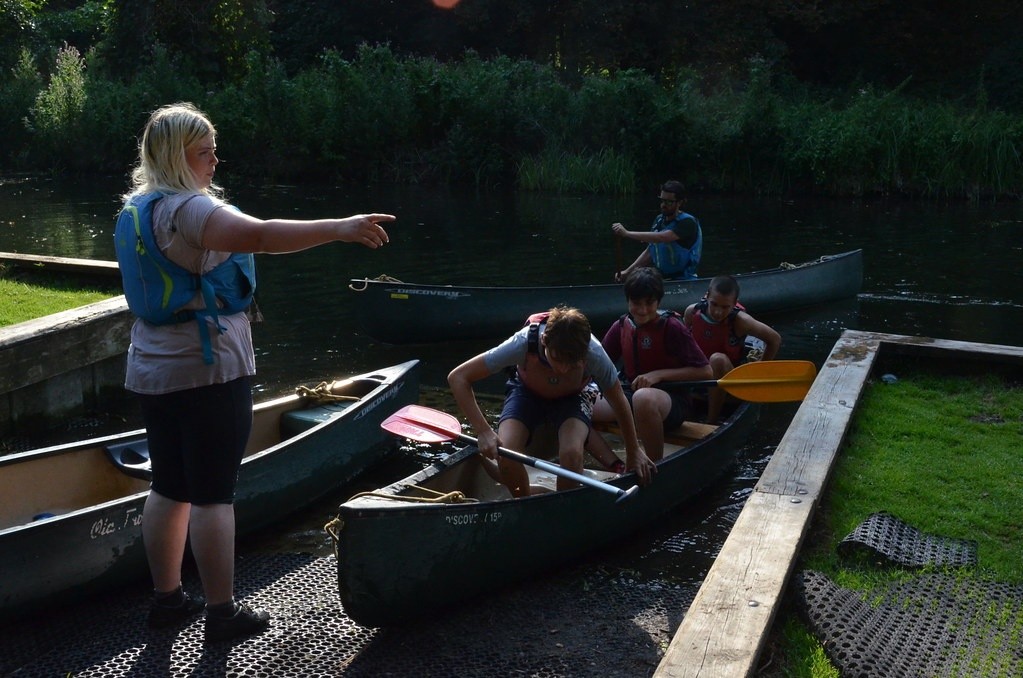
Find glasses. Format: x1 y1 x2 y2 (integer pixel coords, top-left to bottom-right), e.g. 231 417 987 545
657 197 677 204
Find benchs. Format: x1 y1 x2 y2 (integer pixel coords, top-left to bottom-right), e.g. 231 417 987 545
102 437 152 482
594 420 721 448
476 450 621 492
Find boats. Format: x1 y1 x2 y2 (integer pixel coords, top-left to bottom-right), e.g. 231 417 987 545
340 246 870 349
330 392 770 631
0 355 428 605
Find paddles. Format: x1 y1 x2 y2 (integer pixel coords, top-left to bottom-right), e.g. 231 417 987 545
379 402 641 507
618 359 818 404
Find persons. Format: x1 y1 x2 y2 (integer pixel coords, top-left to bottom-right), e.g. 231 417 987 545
447 304 658 497
113 103 396 640
613 180 702 282
581 268 781 474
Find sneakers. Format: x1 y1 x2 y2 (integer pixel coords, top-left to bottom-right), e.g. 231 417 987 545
205 602 270 638
141 590 203 621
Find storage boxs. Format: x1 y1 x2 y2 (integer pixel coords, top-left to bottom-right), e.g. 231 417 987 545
279 400 356 443
